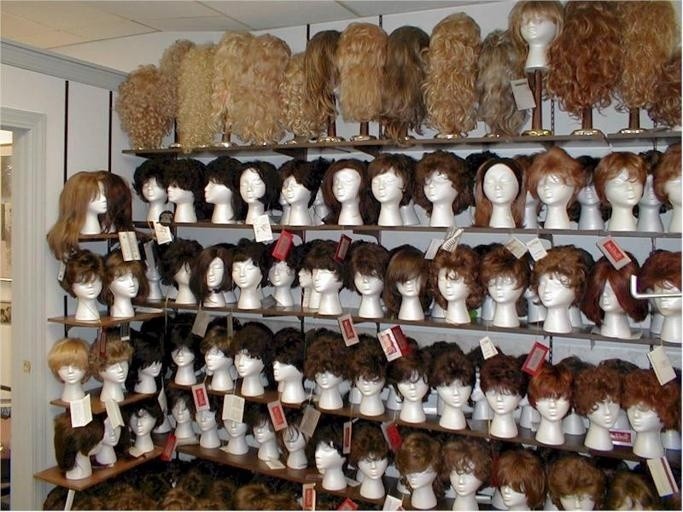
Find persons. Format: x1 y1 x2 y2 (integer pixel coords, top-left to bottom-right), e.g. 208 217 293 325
42 0 681 511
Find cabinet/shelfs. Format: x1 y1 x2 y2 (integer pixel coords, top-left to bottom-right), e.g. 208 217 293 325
122 132 682 511
33 232 172 511
